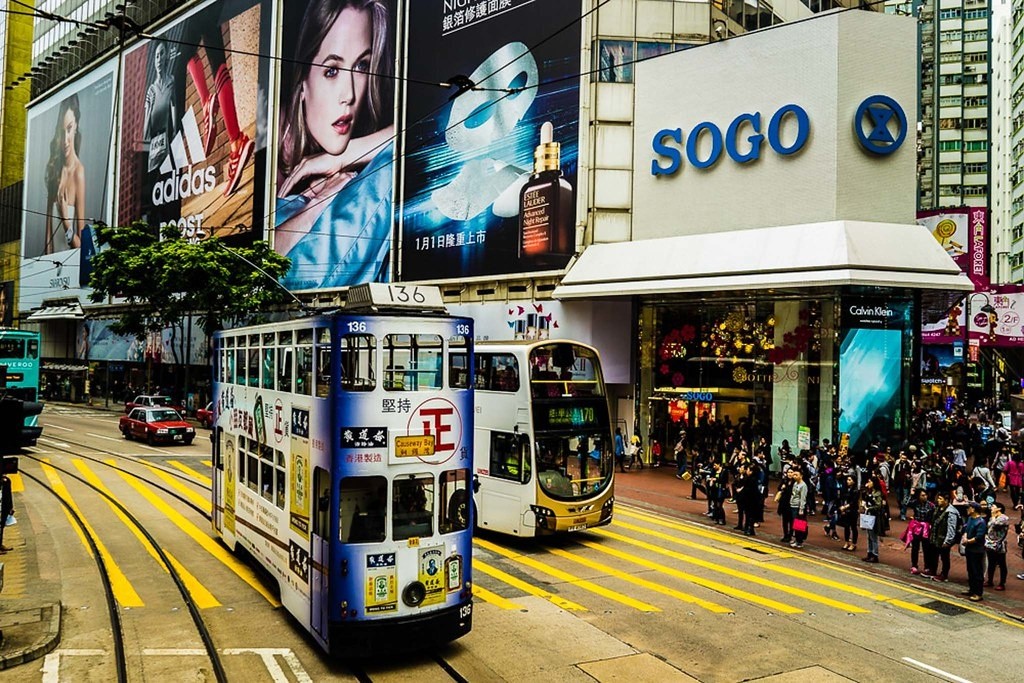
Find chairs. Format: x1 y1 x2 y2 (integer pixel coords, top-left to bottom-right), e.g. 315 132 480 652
385 365 405 388
541 370 560 397
560 372 577 396
492 365 517 392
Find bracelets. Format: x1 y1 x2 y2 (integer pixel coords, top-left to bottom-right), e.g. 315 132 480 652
65 227 73 243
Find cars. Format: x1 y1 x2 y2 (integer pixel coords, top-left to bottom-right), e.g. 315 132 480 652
196 397 214 428
118 404 196 446
125 395 188 420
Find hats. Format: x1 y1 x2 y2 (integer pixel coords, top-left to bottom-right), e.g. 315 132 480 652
876 452 885 461
679 430 686 434
969 501 982 511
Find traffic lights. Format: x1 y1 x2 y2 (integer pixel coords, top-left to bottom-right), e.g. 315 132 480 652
24 399 44 446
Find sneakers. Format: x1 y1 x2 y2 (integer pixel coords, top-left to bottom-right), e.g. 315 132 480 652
222 131 255 198
202 92 220 158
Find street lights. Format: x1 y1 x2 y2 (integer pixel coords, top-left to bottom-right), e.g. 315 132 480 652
959 292 995 419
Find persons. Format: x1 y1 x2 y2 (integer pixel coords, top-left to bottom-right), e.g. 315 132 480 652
135 4 254 230
38 365 212 410
613 392 1024 604
274 0 395 257
42 95 87 253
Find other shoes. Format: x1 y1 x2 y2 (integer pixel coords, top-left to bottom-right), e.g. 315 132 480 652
841 542 850 549
920 568 935 579
983 581 993 587
862 553 873 561
726 498 736 504
0 545 13 551
702 509 804 549
866 554 878 563
831 533 841 541
910 567 918 574
848 543 856 551
961 589 970 594
995 584 1005 591
824 525 830 536
969 594 983 602
1017 573 1024 580
932 574 949 583
0 551 6 555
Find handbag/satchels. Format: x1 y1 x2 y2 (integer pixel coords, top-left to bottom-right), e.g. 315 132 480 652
5 514 17 525
860 508 875 530
999 472 1006 487
792 515 807 532
958 538 967 556
630 445 639 454
774 491 782 502
682 471 692 481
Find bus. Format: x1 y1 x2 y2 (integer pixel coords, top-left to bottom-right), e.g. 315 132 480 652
0 328 42 428
209 237 481 662
283 337 629 540
